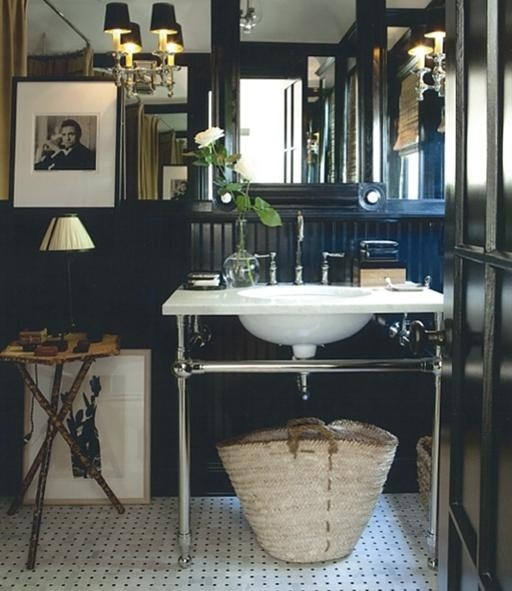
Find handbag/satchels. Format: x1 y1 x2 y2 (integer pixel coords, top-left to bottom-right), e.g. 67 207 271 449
213 416 401 568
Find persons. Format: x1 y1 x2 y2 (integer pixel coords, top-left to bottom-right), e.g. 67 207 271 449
172 181 187 200
39 118 96 171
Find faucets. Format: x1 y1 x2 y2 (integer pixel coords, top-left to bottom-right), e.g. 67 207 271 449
294 210 305 286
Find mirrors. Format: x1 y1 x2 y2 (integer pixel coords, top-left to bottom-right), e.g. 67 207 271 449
0 0 446 216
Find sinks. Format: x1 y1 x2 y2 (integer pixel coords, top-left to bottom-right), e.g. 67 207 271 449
238 285 373 347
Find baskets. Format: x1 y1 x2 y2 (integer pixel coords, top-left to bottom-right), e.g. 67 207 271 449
415 434 434 522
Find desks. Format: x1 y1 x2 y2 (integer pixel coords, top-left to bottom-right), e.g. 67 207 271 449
0 333 126 571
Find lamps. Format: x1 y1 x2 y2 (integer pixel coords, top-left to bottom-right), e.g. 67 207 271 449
424 30 444 98
37 212 97 337
122 22 184 97
102 2 177 87
407 44 433 101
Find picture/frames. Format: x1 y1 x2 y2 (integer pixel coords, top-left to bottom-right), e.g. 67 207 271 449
20 347 152 505
157 163 190 202
7 76 120 211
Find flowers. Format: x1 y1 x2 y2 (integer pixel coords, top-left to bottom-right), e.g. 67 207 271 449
182 128 283 286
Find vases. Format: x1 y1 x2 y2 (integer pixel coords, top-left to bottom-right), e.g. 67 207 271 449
223 250 262 289
